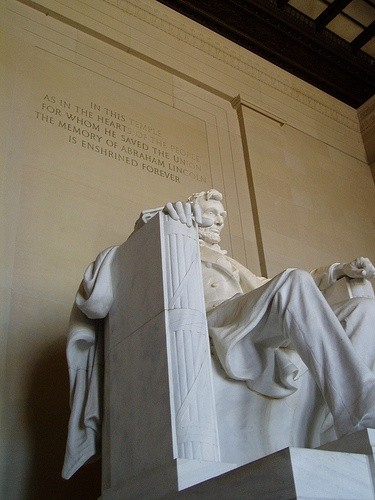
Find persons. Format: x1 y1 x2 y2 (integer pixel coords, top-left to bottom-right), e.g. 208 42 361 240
159 188 375 446
